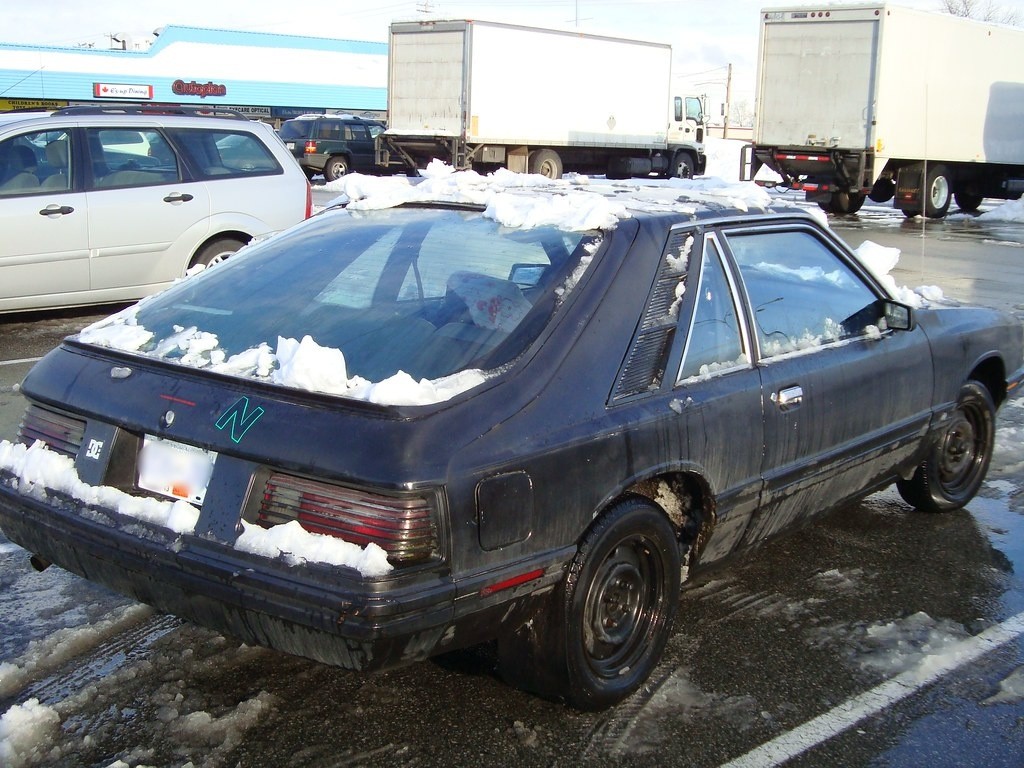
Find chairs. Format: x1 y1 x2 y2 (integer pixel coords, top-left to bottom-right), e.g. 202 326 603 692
40 140 69 190
443 268 533 332
0 145 40 191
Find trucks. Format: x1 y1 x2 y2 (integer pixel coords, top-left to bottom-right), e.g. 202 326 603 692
739 5 1024 218
384 20 711 180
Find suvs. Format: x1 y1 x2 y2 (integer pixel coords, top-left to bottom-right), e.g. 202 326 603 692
280 111 407 182
0 104 312 314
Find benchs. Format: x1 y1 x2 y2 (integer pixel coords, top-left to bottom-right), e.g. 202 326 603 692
96 169 179 187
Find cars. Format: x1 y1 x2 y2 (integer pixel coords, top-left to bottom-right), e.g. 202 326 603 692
0 179 1024 716
